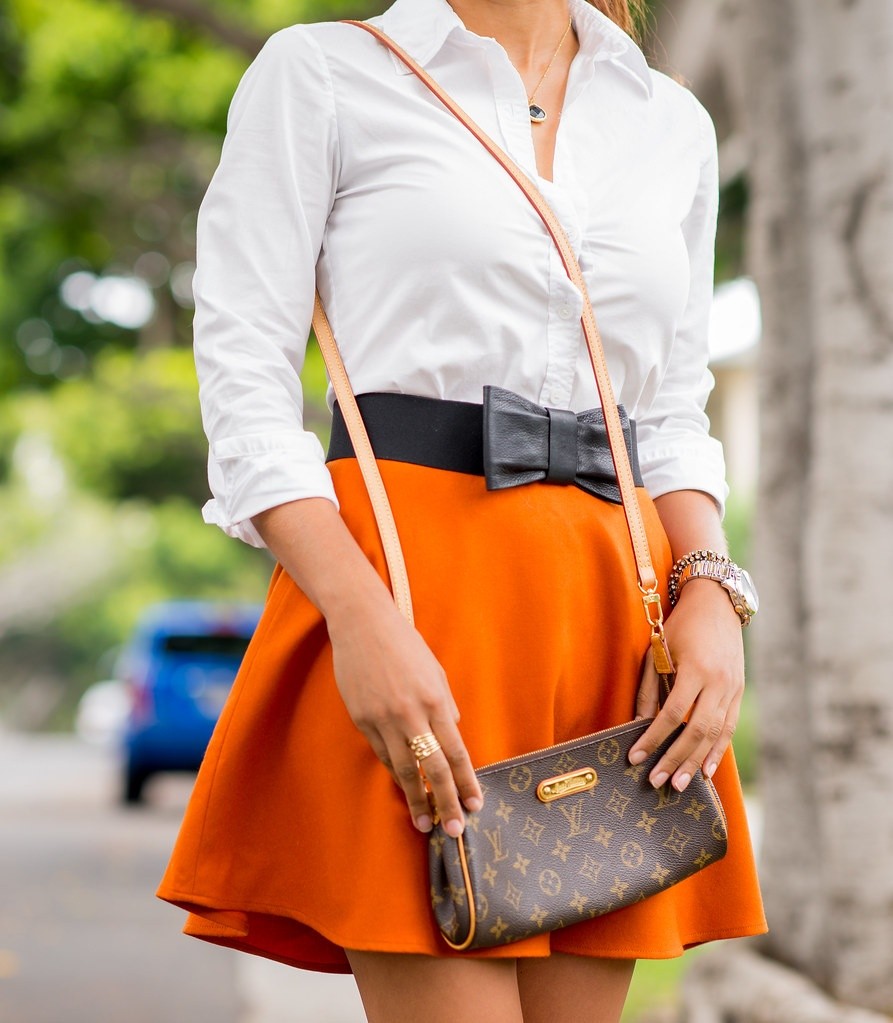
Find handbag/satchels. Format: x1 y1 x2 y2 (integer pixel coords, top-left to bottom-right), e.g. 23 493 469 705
428 714 730 952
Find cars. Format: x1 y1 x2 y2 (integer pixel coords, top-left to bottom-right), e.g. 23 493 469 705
105 604 276 802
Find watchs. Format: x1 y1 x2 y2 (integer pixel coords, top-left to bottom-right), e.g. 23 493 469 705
675 561 759 627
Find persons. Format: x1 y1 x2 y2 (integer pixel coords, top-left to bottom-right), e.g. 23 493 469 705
156 0 770 1023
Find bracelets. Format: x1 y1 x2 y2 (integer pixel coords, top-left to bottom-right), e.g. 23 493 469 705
669 550 738 607
408 732 441 760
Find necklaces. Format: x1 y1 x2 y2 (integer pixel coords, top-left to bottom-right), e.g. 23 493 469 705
528 16 573 121
